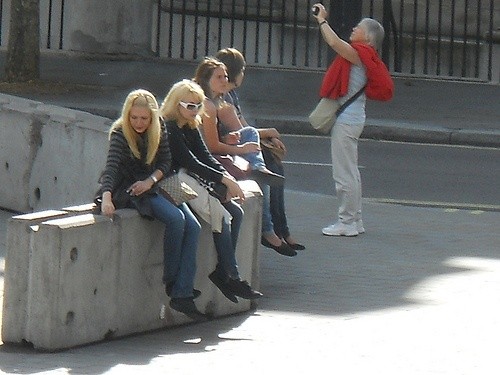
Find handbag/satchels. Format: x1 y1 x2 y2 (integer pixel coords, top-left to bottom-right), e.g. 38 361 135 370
157 174 199 208
308 96 340 135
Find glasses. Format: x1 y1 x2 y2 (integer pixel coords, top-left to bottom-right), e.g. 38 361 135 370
179 101 202 111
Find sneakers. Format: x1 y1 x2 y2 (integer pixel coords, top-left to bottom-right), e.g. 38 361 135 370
321 224 365 237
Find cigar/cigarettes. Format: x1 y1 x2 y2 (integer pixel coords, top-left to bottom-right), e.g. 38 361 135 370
242 198 245 200
109 218 113 222
257 147 262 151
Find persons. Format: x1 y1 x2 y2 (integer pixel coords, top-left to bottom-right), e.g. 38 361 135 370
159 80 263 302
312 3 384 236
196 58 297 258
215 47 307 250
95 88 209 322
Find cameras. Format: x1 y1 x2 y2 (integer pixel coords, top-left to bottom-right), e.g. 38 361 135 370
311 5 319 15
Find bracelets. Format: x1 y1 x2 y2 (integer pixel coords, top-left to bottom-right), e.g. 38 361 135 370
151 174 158 184
319 20 328 27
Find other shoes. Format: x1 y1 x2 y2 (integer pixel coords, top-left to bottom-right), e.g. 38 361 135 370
169 296 208 321
209 271 237 303
231 279 263 298
253 169 285 187
261 236 297 256
288 242 304 250
166 284 201 299
